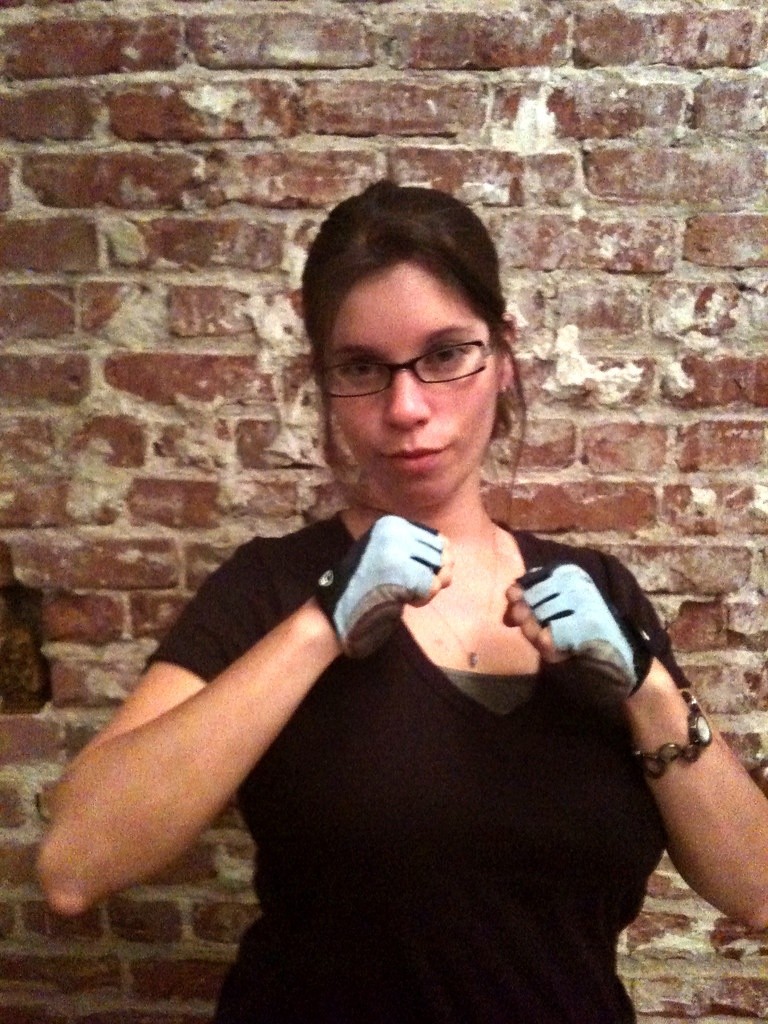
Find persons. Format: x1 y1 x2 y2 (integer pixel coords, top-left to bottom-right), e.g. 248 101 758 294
36 179 768 1024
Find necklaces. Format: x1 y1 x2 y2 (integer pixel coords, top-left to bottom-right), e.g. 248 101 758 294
428 530 499 668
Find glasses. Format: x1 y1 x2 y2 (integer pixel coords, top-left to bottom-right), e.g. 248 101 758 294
313 339 494 398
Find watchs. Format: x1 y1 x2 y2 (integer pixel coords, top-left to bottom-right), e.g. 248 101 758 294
630 690 712 779
